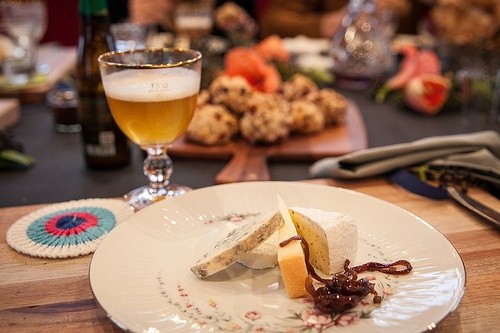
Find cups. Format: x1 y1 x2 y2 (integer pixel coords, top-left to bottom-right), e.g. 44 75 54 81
109 21 149 52
47 86 81 134
2 42 37 87
169 0 215 67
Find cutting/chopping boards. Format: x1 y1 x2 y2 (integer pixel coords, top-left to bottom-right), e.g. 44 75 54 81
165 91 366 183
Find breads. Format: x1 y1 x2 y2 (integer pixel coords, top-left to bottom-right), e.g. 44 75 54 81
184 74 348 146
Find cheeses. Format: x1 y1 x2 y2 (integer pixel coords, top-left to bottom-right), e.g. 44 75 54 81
277 194 312 298
189 211 284 279
288 207 358 274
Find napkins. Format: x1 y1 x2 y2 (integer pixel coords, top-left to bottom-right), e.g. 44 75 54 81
310 130 500 177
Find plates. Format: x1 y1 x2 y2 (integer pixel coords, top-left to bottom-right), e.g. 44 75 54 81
0 44 77 104
90 181 466 333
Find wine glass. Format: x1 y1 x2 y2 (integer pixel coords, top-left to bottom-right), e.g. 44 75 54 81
1 0 46 77
96 47 201 213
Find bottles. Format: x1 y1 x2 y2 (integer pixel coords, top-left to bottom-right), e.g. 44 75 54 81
77 0 132 171
329 0 397 80
